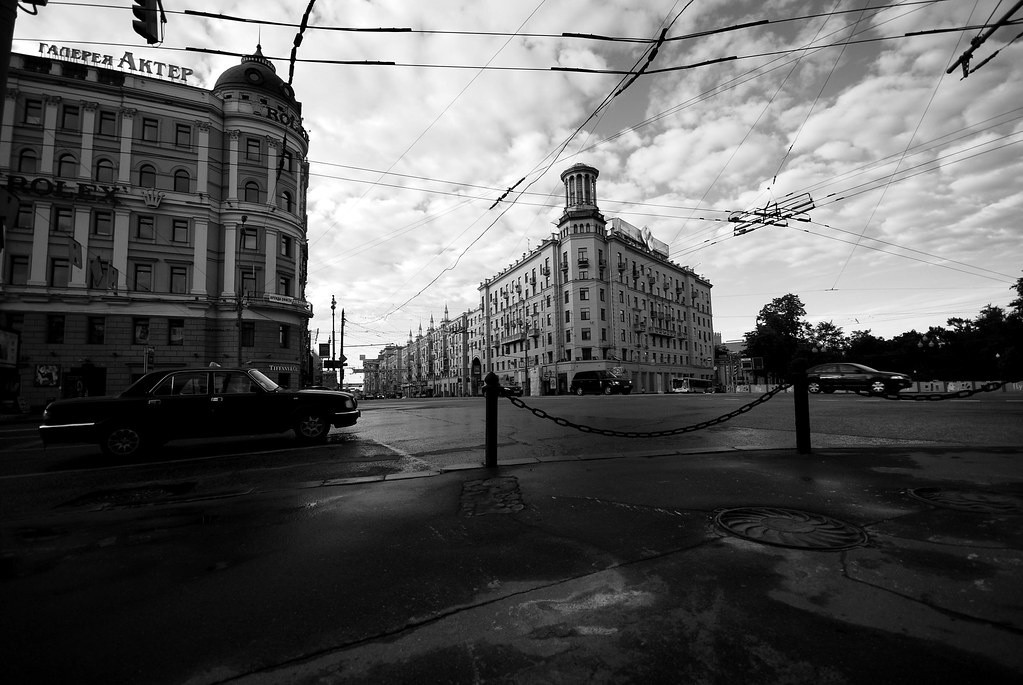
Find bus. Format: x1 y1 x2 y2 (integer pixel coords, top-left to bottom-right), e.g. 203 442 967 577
671 376 714 393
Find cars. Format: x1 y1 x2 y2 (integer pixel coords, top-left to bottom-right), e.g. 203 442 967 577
482 380 523 397
805 362 912 397
360 389 386 400
38 361 361 465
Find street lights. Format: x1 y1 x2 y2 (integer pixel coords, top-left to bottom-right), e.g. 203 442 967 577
520 321 532 396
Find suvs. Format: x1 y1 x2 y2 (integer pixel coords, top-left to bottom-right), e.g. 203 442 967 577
570 369 633 395
